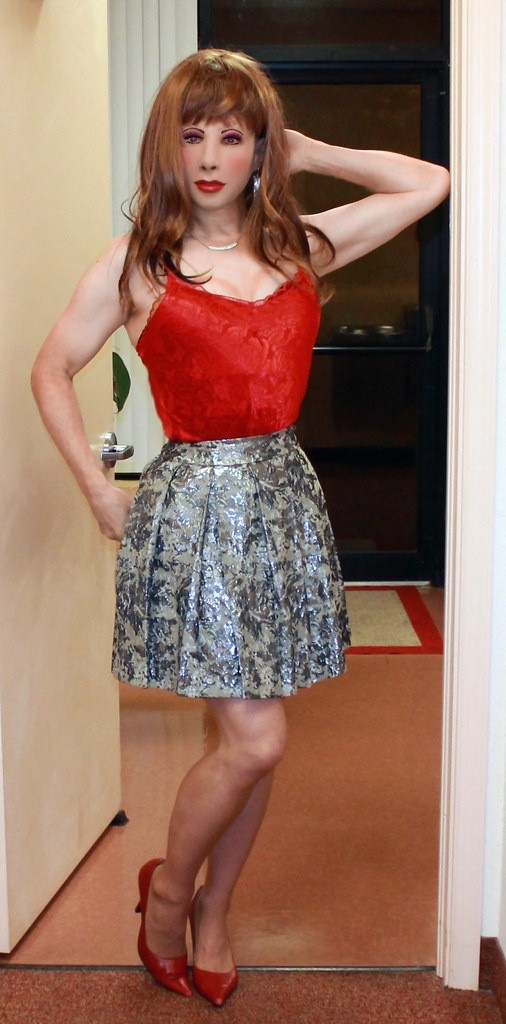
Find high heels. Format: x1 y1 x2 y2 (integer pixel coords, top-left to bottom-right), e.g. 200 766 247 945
188 884 239 1005
134 858 194 998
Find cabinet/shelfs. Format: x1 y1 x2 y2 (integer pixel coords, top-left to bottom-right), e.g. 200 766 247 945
295 344 416 448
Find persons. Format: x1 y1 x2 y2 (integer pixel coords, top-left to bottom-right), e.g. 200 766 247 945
29 47 455 1007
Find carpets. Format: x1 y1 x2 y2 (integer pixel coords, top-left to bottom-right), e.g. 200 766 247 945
340 585 444 656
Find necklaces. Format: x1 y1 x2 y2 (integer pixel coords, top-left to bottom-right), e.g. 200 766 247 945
187 230 252 252
